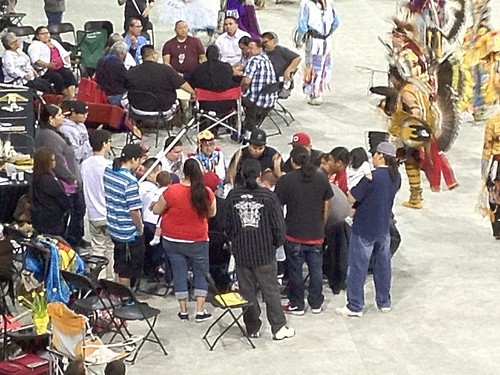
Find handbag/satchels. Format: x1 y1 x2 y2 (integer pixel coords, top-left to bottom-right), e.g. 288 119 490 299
13 194 31 223
0 353 55 375
1 238 16 314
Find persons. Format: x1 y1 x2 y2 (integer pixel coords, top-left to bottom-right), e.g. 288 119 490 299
104 360 127 375
272 146 335 317
218 158 298 341
65 357 88 375
0 0 500 321
295 0 341 107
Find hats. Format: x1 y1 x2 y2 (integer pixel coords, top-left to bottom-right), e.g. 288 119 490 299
288 131 311 146
249 128 267 146
121 142 147 158
371 142 397 157
63 100 89 115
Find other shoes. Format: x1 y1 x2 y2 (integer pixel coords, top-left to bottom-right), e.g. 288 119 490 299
334 305 363 317
173 124 186 133
195 312 213 322
375 300 391 312
402 195 424 208
178 312 189 320
276 261 285 278
85 242 92 249
308 96 322 105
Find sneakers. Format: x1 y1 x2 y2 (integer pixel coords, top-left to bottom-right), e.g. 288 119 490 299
271 326 295 339
311 301 324 313
282 302 304 316
250 331 259 338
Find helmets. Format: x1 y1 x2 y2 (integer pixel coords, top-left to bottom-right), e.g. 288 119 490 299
197 129 215 143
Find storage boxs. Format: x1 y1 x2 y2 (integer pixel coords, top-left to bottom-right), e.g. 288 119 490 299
0 355 49 375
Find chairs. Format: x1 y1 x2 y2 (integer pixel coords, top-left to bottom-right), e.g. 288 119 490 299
0 0 295 375
